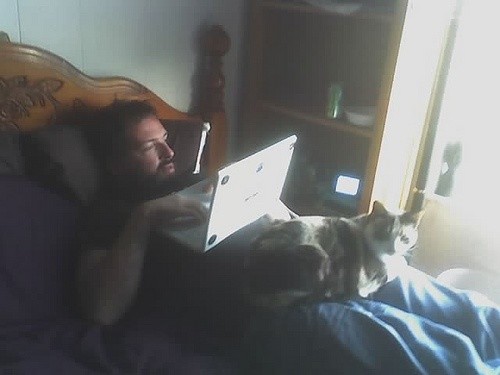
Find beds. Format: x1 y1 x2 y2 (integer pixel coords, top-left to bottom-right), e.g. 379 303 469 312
0 22 500 375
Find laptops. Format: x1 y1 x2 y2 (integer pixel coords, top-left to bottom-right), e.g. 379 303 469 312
142 134 298 255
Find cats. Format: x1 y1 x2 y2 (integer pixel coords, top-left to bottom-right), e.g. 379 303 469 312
235 200 427 321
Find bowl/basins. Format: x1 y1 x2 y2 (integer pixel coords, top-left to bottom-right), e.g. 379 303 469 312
342 104 378 127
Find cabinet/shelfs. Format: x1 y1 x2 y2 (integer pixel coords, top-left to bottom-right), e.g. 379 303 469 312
231 0 458 220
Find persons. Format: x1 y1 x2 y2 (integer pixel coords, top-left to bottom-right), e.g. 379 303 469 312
64 99 500 375
